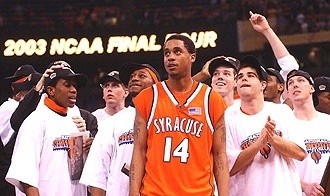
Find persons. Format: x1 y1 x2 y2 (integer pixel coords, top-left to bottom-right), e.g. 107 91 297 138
0 0 330 196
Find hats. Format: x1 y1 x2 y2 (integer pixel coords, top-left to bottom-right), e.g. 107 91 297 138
208 56 285 95
99 62 162 89
286 69 330 93
4 60 87 90
